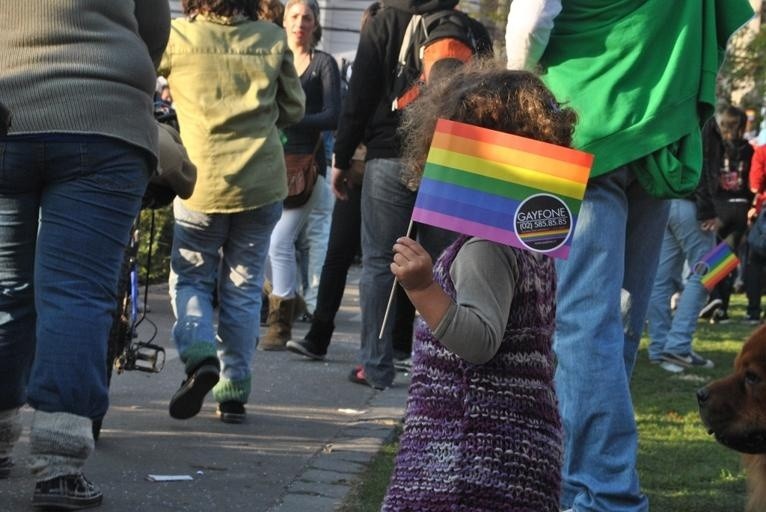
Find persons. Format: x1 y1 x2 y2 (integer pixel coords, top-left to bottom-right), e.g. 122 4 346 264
0 1 170 507
379 54 578 510
149 0 765 422
505 0 753 510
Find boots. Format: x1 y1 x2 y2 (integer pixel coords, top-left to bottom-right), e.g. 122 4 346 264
257 293 295 350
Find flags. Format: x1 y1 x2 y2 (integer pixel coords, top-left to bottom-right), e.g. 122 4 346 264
412 116 597 259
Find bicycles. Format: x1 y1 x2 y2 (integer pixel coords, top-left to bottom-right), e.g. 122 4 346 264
0 99 181 400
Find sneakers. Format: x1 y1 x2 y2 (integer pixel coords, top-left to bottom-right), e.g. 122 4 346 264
657 348 714 369
698 299 731 324
286 338 324 360
349 365 384 390
32 474 103 508
170 358 219 418
739 315 761 325
216 401 246 422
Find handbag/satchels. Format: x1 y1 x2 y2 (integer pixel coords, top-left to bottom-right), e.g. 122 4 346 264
284 153 318 208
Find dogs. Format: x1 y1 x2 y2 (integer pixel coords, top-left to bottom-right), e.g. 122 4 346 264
695 323 766 512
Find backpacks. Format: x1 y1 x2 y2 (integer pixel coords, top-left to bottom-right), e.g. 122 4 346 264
391 10 493 112
